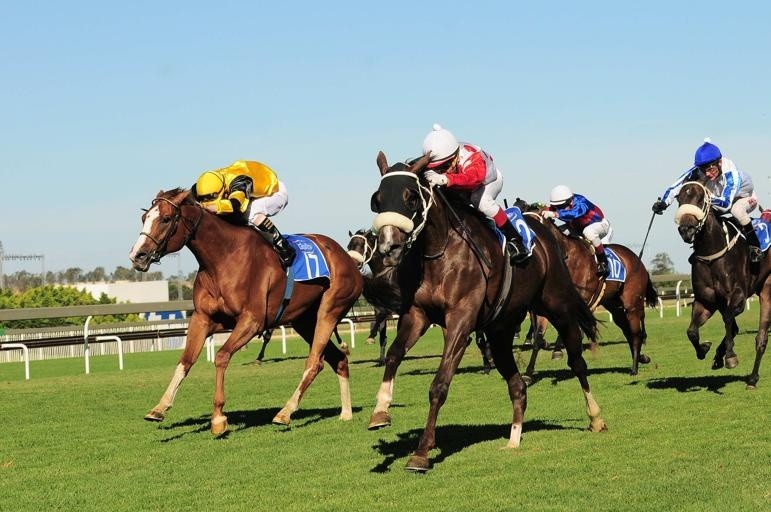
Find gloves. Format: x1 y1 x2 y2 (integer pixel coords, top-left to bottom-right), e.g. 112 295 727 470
652 202 667 215
542 211 555 219
424 170 447 187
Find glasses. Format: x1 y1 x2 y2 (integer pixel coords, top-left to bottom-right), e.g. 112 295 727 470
700 167 718 174
198 193 214 201
435 154 455 170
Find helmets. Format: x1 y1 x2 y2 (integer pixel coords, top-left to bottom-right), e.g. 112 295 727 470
694 142 722 166
196 170 224 203
550 185 572 205
428 131 458 168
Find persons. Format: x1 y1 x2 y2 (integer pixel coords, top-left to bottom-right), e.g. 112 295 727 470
422 122 531 261
190 159 297 266
541 185 613 275
651 136 765 263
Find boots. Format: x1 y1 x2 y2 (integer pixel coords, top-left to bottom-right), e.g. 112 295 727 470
500 217 527 263
595 250 610 277
257 218 295 267
741 220 761 271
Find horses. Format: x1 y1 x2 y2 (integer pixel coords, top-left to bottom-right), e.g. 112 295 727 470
371 148 608 472
347 229 492 374
677 175 771 390
522 204 657 387
128 184 401 437
